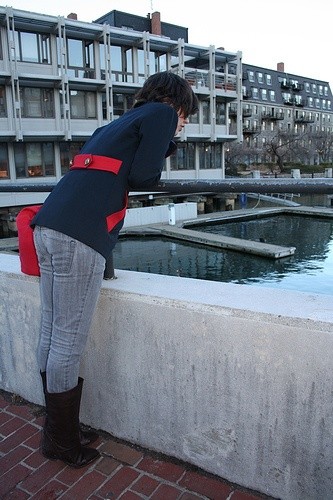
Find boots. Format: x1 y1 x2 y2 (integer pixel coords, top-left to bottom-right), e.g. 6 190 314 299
39 368 99 447
41 376 102 469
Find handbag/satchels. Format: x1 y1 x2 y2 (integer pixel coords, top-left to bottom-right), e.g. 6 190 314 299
15 203 43 277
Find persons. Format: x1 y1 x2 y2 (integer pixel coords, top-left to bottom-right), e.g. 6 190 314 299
29 74 198 467
